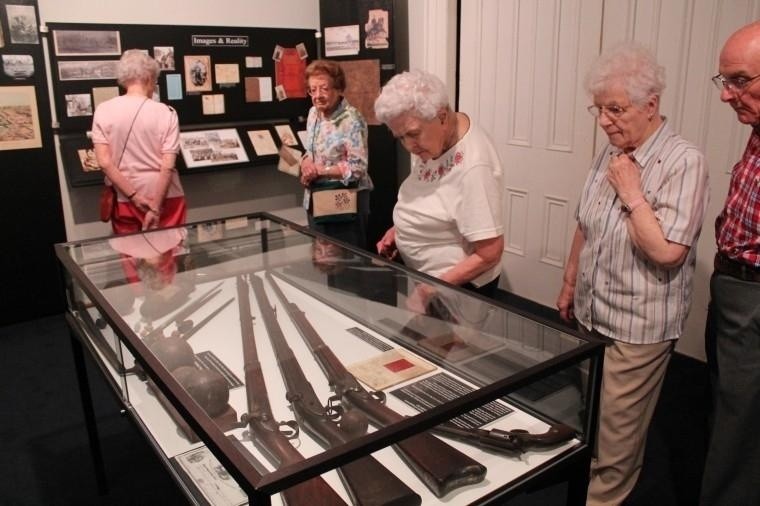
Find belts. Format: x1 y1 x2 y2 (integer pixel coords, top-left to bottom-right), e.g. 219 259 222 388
714 253 760 283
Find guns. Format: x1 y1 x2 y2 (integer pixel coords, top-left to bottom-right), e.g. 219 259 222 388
235 271 348 506
249 274 423 503
265 270 486 497
431 422 568 457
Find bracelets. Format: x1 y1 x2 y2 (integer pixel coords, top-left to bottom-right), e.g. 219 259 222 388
121 187 142 202
324 164 331 179
615 196 651 214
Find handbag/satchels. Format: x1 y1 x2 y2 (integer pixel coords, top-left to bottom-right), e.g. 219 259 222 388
99 186 113 222
310 180 359 225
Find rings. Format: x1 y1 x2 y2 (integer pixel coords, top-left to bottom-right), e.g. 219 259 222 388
301 180 308 185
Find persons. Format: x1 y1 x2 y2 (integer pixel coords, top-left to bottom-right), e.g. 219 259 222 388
108 225 193 291
310 235 347 276
389 286 491 369
554 40 711 504
703 21 760 504
376 67 506 327
297 59 375 245
92 49 186 288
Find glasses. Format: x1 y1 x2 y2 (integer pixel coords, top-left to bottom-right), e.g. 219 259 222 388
307 86 334 97
712 74 760 92
588 105 632 118
